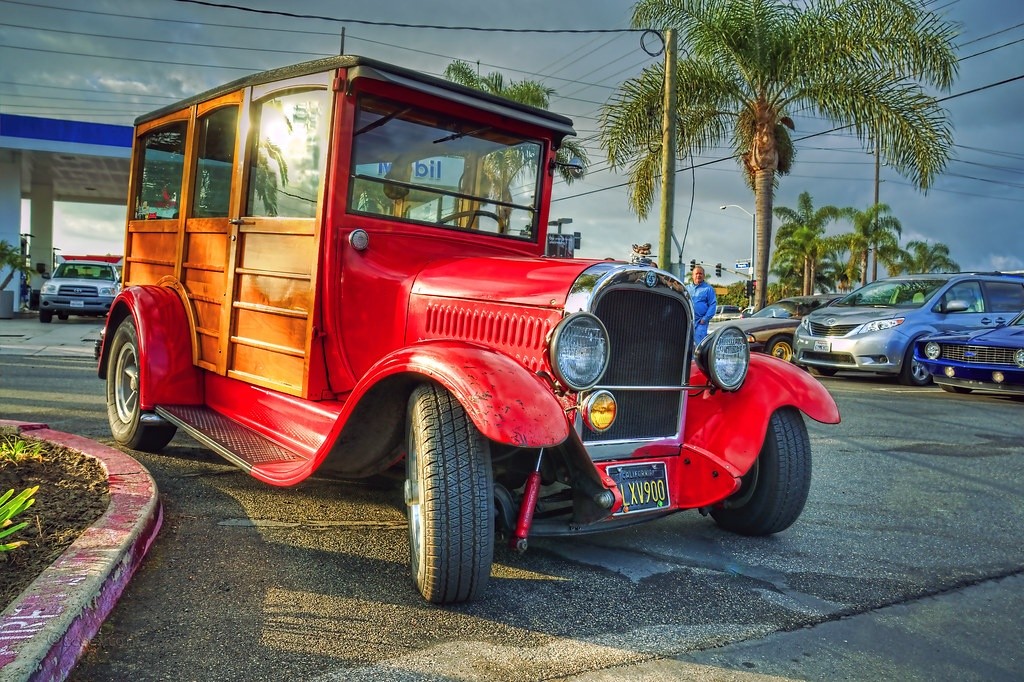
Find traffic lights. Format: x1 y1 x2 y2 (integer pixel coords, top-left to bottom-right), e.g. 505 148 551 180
715 263 722 278
751 280 757 295
690 259 695 272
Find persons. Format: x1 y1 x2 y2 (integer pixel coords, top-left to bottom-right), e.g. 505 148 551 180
685 266 717 348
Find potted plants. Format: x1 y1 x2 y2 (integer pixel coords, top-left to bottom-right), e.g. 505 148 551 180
0 241 37 319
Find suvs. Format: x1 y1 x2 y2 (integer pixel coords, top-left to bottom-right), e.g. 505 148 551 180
39 259 122 323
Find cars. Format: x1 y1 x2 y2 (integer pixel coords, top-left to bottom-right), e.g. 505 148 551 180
98 54 840 604
707 295 849 364
913 310 1024 396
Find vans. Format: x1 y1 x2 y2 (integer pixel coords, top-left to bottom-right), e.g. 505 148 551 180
792 271 978 386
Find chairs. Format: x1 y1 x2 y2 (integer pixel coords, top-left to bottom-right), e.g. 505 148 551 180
64 268 79 278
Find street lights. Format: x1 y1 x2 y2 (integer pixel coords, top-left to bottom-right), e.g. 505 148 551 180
720 205 755 306
